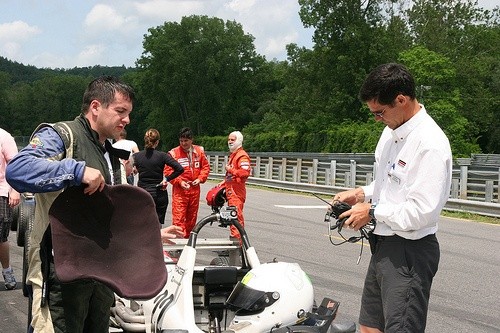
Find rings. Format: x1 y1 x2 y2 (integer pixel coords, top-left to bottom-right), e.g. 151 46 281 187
350 222 355 227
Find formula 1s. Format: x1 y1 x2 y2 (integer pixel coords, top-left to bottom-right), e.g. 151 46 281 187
12 192 43 298
87 206 356 333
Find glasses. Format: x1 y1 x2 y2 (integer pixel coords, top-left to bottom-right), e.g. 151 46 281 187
372 105 391 118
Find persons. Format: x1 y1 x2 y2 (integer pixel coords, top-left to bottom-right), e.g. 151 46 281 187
0 79 210 333
216 131 251 245
331 63 453 333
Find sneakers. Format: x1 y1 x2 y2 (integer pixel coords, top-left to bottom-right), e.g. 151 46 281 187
2 267 17 290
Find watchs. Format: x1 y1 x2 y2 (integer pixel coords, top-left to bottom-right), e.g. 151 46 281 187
369 203 376 217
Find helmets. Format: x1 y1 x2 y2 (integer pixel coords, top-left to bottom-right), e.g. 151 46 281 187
226 262 314 333
206 182 227 207
